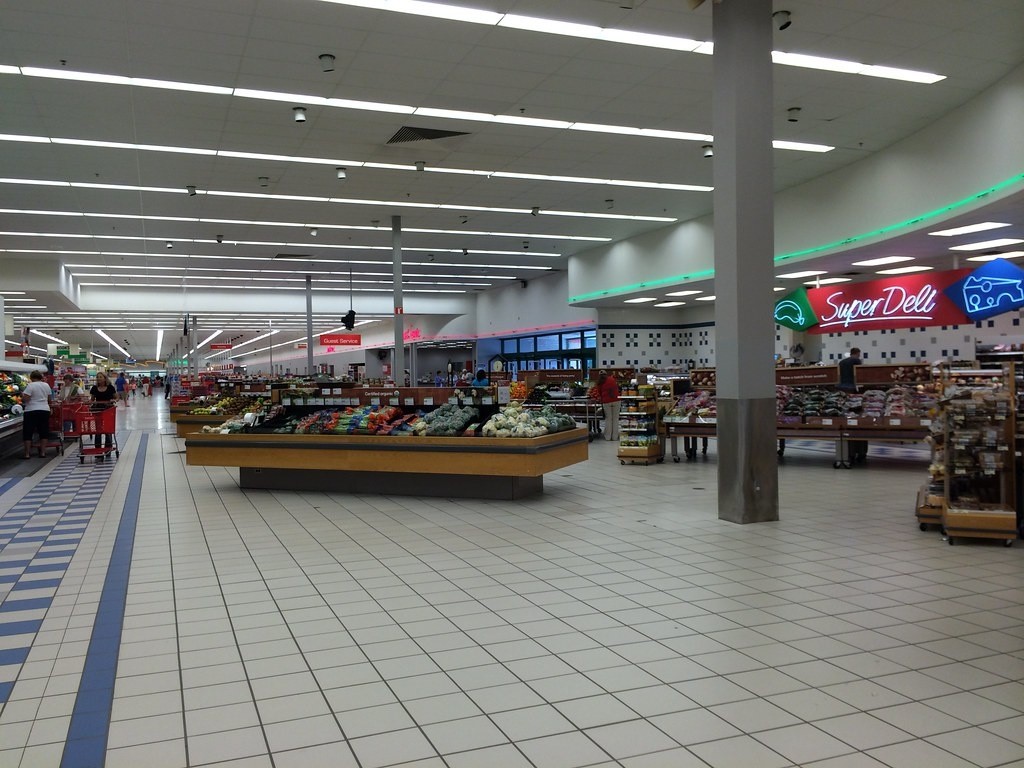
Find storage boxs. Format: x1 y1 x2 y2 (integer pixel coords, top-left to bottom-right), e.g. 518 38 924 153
778 416 804 424
840 415 883 427
883 416 917 428
917 417 938 427
806 415 840 424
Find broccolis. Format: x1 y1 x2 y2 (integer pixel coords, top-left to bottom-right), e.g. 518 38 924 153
482 401 577 437
416 402 478 436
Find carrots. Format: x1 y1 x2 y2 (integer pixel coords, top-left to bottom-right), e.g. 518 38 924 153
376 413 415 434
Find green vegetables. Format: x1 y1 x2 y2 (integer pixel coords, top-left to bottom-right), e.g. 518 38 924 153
0 373 27 410
274 405 396 432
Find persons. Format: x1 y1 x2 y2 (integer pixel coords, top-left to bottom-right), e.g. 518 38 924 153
472 370 488 386
839 347 862 388
156 374 161 382
164 381 171 399
434 371 444 387
446 368 473 382
115 373 130 407
22 370 53 460
404 370 410 378
130 381 137 397
89 372 120 463
597 370 621 441
60 375 84 431
142 376 152 396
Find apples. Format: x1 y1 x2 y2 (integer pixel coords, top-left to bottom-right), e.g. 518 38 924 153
186 397 237 415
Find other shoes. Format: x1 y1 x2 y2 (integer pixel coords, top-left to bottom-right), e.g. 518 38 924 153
40 452 45 457
94 458 101 463
16 454 30 459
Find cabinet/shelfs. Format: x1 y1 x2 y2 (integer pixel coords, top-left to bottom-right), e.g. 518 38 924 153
914 336 1024 547
615 382 666 466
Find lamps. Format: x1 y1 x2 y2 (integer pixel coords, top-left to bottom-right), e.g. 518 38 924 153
787 109 799 123
259 177 268 188
295 109 307 123
187 186 196 197
774 12 791 30
459 217 469 224
337 168 347 180
216 235 224 243
531 207 541 216
463 249 468 256
428 255 434 261
523 241 530 248
606 201 614 209
372 220 380 228
167 242 173 250
310 229 318 237
702 145 714 159
321 55 336 73
416 162 424 172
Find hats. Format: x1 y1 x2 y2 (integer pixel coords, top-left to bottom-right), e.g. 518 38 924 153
63 374 75 381
599 370 607 375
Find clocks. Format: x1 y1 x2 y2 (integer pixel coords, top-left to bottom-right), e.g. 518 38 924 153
493 360 503 372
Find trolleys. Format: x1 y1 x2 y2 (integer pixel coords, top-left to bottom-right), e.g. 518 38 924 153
148 386 153 396
31 401 64 457
75 401 119 464
61 396 92 443
